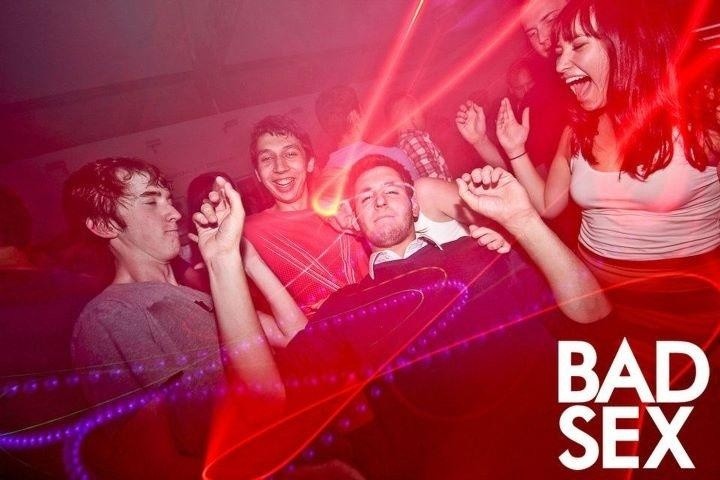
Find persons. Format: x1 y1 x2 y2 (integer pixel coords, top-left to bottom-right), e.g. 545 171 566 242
504 51 534 105
496 2 719 311
306 81 417 202
1 195 82 368
62 157 311 429
238 115 372 319
171 166 246 292
386 92 458 182
192 156 614 405
456 2 574 178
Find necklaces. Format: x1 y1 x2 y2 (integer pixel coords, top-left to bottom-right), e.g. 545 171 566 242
509 149 529 161
